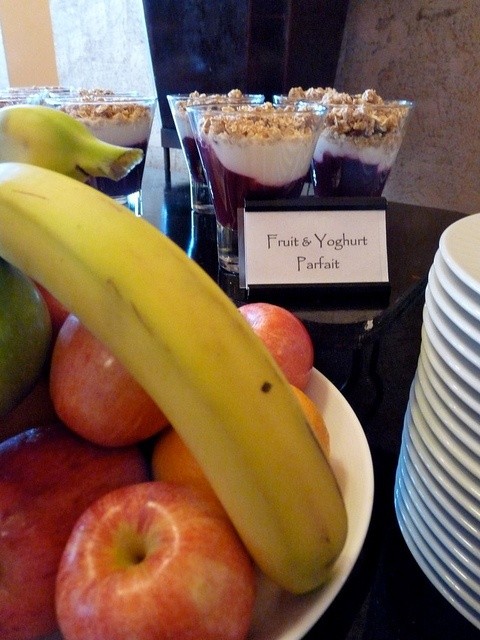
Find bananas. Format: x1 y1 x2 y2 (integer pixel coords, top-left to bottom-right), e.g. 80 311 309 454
1 104 144 184
0 167 350 595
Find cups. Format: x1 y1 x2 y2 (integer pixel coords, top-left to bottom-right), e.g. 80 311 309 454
44 98 159 218
165 93 265 215
50 89 141 97
274 95 315 197
186 103 330 278
0 85 71 107
294 94 417 199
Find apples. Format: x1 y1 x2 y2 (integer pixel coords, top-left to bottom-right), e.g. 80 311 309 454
240 302 314 390
54 480 254 639
49 315 167 443
151 384 332 510
0 424 151 640
0 260 50 419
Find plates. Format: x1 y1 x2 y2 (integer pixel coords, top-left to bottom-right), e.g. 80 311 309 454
391 213 480 632
242 357 379 640
